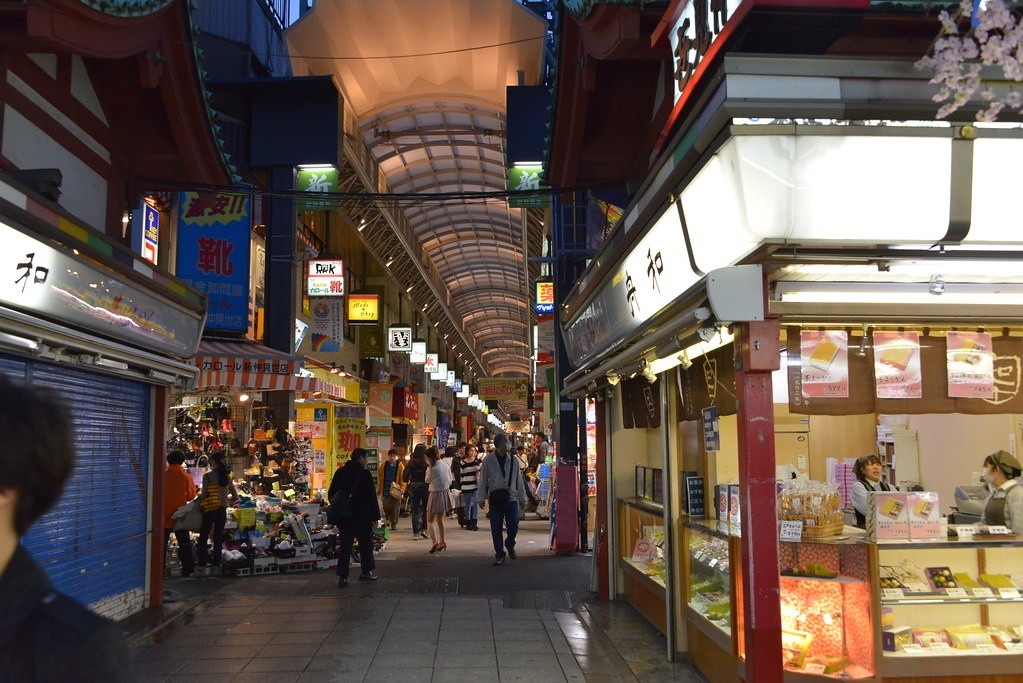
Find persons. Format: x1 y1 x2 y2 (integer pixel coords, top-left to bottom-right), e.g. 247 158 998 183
850 454 898 531
481 438 495 461
163 450 197 576
442 442 481 531
401 444 430 539
189 452 238 576
377 449 406 530
529 432 549 477
424 446 452 553
0 370 131 683
328 448 381 585
513 446 529 479
973 450 1023 535
479 434 527 565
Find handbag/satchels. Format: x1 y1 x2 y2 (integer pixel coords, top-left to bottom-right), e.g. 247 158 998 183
327 496 355 526
452 489 474 507
390 481 403 499
189 455 213 485
489 489 510 505
200 473 220 513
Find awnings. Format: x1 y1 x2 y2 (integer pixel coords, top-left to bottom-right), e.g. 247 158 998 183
191 337 368 401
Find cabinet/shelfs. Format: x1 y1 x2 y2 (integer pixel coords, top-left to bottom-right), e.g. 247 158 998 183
618 496 667 638
677 516 745 682
857 532 1023 683
779 538 876 682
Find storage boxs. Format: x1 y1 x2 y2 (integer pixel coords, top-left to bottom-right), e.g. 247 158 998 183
223 504 334 566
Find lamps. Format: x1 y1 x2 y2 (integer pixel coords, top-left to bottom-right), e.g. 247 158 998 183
655 336 681 358
855 325 871 358
587 396 594 405
0 332 180 385
642 363 658 383
607 388 615 398
616 358 646 377
313 391 322 396
238 386 249 402
596 393 603 402
929 275 947 293
677 348 693 370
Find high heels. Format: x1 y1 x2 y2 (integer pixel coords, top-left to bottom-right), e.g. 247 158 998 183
429 542 440 553
438 543 446 551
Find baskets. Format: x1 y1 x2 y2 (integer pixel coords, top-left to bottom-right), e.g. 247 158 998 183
801 521 844 537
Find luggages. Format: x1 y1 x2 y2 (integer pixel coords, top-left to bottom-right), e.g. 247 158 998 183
458 492 473 527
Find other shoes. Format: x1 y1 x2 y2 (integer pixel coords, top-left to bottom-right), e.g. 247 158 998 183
414 533 419 539
169 398 311 485
339 579 348 586
508 549 516 559
467 527 472 530
474 526 479 531
359 571 378 580
392 527 396 530
421 529 430 539
495 557 505 565
387 524 389 526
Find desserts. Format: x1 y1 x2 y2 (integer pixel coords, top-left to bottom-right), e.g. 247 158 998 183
930 570 955 589
879 578 904 589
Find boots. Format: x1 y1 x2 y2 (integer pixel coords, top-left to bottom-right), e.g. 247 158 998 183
206 565 221 577
189 566 206 577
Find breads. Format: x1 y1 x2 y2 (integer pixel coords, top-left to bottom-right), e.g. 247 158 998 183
810 337 841 371
880 343 914 371
